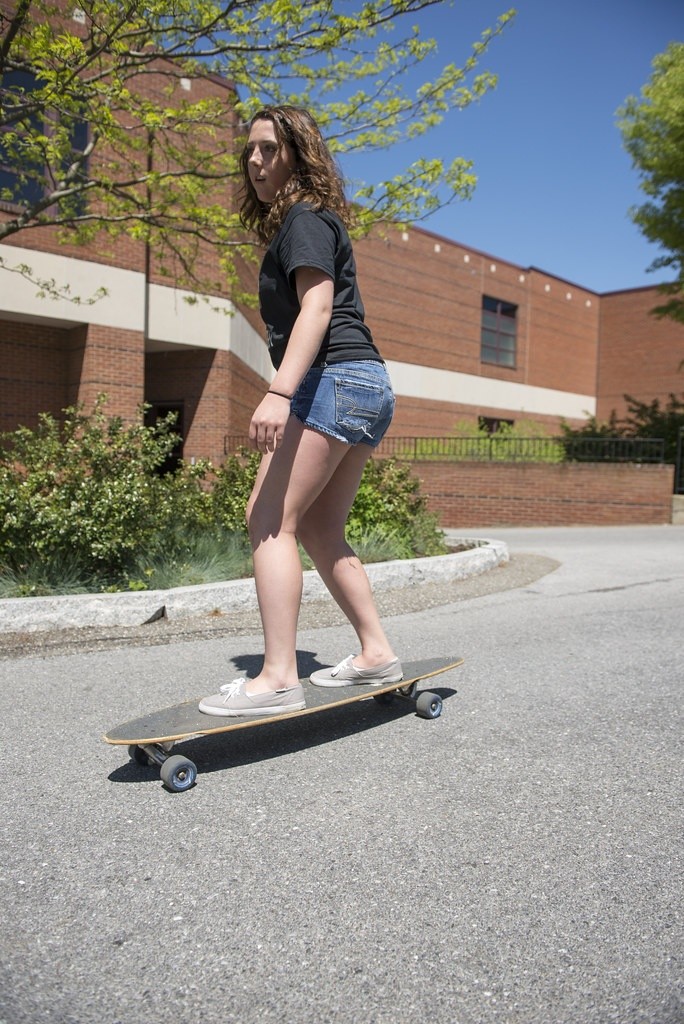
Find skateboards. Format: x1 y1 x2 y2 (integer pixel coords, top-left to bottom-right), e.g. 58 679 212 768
105 655 466 793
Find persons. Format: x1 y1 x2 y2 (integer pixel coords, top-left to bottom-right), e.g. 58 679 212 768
197 104 403 716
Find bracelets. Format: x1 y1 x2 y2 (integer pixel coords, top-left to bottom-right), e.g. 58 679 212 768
266 388 295 402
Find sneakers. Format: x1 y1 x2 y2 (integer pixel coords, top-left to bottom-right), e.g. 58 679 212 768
199 678 306 716
310 654 403 687
